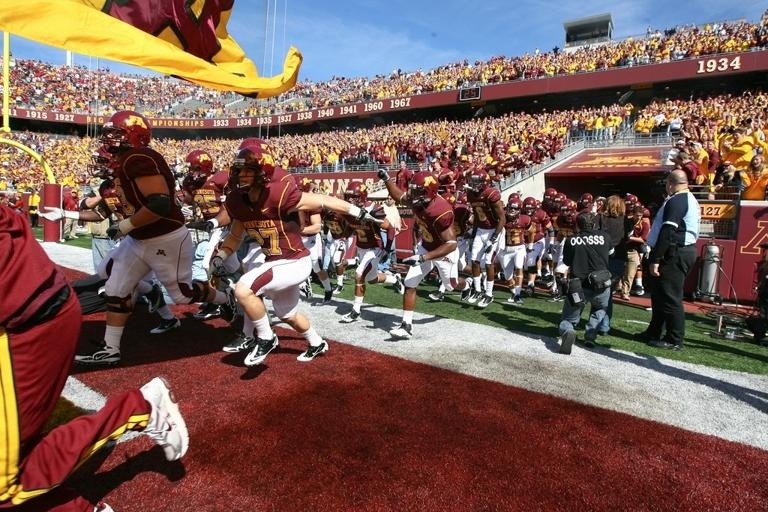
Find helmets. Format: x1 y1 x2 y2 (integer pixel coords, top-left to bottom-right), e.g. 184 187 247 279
85 110 651 218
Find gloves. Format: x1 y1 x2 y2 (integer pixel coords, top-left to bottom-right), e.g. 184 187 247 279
484 240 493 254
106 225 123 241
402 254 425 268
206 255 224 286
546 244 554 254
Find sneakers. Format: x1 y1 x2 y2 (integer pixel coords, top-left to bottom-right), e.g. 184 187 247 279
138 374 191 464
74 273 683 368
